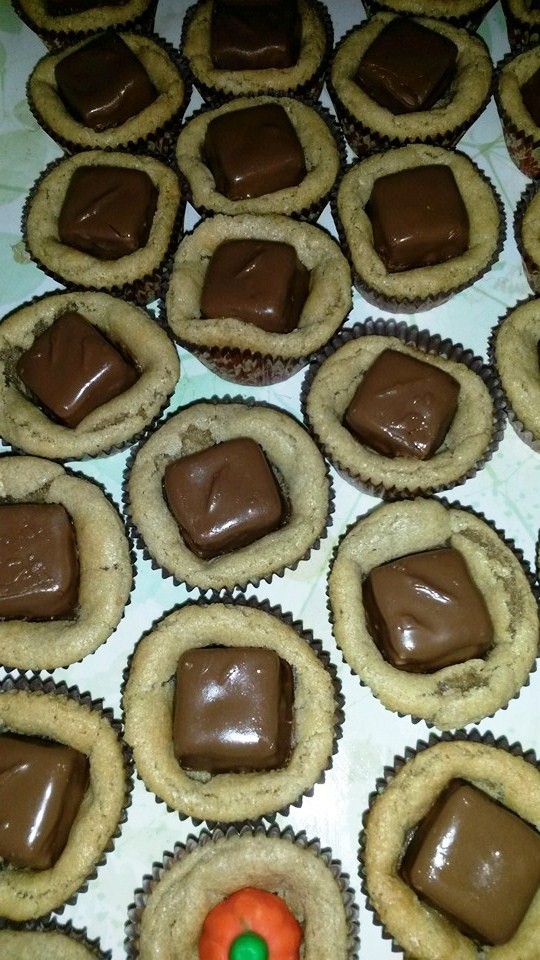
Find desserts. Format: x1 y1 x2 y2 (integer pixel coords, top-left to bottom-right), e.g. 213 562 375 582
2 5 540 959
